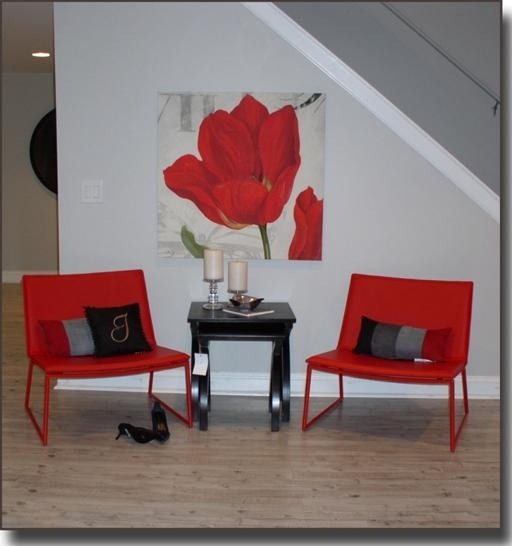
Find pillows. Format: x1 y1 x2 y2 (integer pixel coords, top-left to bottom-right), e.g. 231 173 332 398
352 314 453 362
37 301 154 360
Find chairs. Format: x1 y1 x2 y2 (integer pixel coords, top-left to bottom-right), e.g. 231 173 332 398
301 274 473 453
22 270 192 446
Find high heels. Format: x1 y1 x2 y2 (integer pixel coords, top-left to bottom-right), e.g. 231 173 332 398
115 423 156 444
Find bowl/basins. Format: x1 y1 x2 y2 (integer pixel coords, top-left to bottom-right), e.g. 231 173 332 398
229 295 265 312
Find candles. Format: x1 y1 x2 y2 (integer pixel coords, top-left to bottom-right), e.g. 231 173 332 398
203 248 248 291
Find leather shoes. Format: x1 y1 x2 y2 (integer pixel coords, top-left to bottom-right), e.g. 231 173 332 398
150 400 171 443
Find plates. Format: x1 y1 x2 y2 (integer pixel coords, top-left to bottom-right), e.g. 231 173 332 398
221 308 275 318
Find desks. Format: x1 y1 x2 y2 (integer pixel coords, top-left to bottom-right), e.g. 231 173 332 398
186 301 297 433
